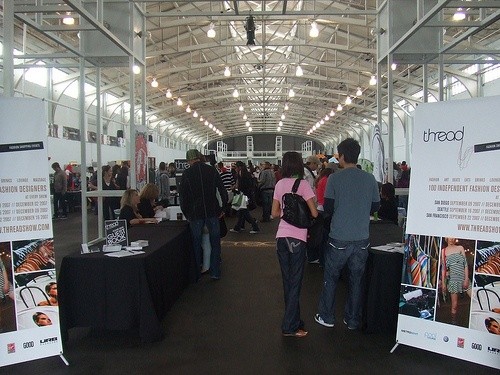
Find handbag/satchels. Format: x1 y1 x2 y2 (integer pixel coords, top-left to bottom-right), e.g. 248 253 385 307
232 189 249 210
282 178 313 228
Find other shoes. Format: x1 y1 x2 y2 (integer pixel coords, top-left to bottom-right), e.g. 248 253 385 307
249 230 260 233
314 312 334 327
228 228 237 232
344 318 355 329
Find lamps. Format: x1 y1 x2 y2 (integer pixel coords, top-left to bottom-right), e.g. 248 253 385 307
62 7 466 136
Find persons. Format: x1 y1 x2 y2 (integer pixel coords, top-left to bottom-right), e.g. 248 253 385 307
38 282 58 306
49 148 410 284
442 237 472 316
485 317 500 335
271 152 318 336
0 256 9 292
33 312 52 326
314 139 380 329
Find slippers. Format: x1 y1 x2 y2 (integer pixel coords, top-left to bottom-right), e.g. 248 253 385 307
282 328 309 337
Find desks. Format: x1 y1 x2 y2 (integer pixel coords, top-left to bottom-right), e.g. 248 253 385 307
359 242 405 336
59 218 198 345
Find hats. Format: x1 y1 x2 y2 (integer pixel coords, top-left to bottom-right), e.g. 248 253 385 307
186 149 200 160
307 155 322 165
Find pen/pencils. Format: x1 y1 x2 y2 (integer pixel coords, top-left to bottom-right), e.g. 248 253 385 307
126 249 135 253
388 247 395 250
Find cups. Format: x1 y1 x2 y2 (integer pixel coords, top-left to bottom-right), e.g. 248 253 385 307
177 212 183 221
